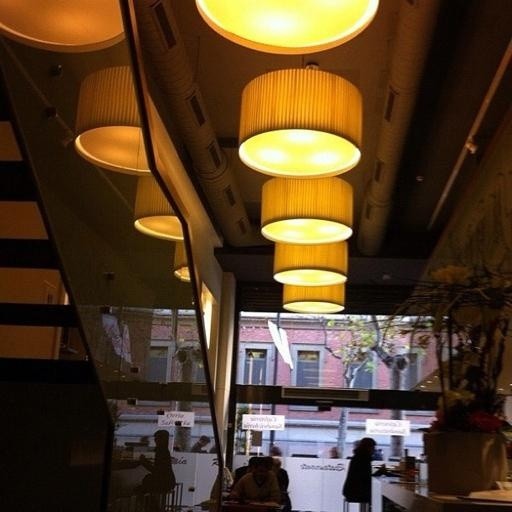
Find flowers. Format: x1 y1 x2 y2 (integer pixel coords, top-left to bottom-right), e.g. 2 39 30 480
416 260 512 435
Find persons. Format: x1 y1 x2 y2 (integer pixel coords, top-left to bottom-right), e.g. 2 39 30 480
272 458 291 512
230 460 282 508
191 435 210 453
236 458 258 480
139 430 175 493
343 438 386 512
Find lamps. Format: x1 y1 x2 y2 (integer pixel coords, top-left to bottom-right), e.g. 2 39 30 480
1 1 126 54
134 175 185 241
260 177 354 313
238 64 362 178
73 63 154 176
198 0 379 55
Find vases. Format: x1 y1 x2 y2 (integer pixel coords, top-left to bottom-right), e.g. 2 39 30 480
422 432 504 496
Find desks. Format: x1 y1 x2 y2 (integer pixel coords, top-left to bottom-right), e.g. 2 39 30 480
378 477 512 511
221 500 287 512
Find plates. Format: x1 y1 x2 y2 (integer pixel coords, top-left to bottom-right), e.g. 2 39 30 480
390 480 416 484
224 500 250 505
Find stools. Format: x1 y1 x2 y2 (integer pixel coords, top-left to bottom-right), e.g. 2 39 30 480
342 497 369 512
109 481 183 512
